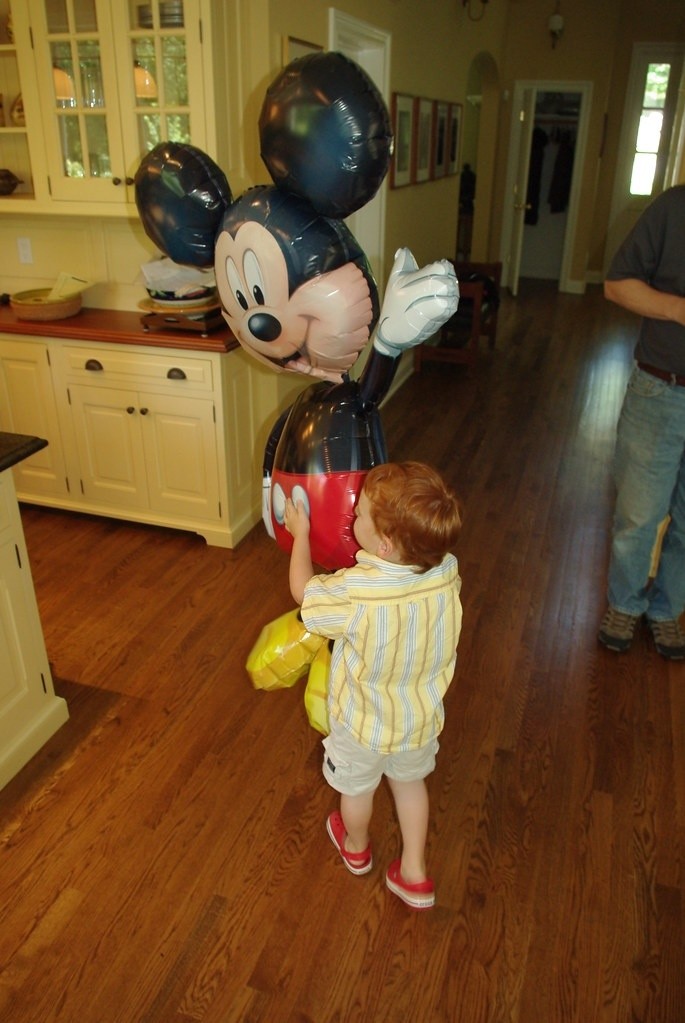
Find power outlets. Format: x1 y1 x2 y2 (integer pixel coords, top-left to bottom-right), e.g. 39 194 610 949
16 235 33 265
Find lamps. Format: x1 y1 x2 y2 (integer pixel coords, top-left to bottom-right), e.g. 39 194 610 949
53 62 76 99
134 60 158 98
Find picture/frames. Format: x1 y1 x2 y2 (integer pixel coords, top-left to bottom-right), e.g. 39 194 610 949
388 90 416 191
431 99 447 182
447 102 464 177
414 96 435 185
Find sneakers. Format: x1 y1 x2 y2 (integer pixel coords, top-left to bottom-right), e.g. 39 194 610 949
600 607 636 653
646 613 685 661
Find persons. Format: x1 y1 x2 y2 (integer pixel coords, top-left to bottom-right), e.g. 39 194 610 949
282 460 462 910
597 180 685 661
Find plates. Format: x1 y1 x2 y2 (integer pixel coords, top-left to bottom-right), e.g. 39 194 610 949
9 92 25 127
138 2 184 28
137 298 221 315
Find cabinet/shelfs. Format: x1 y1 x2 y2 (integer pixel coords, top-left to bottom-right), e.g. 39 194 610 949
0 0 46 214
0 331 74 511
27 0 258 219
412 261 503 384
50 337 283 562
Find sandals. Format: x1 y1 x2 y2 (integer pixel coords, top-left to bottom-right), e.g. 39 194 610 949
386 859 434 908
326 812 372 876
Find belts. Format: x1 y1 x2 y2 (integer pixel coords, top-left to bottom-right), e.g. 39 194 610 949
637 359 685 390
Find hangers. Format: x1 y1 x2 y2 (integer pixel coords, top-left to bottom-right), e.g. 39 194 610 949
549 123 576 145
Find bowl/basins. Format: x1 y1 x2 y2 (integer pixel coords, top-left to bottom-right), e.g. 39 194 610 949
9 289 82 321
144 269 216 307
